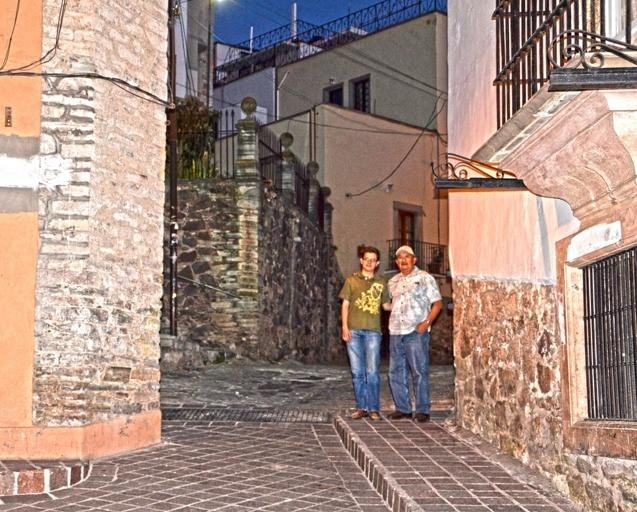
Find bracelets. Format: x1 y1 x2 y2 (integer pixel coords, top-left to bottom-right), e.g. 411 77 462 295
424 319 433 326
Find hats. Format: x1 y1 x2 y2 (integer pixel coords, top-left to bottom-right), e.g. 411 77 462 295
396 245 414 256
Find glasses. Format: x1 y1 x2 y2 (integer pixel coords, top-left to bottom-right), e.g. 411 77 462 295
362 258 375 262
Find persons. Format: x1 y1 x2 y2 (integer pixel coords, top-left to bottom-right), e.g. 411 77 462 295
384 245 442 423
337 245 391 421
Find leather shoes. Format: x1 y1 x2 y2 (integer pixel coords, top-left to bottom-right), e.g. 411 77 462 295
388 411 412 420
414 413 431 423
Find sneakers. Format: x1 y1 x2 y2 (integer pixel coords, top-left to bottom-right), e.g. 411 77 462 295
351 410 368 418
370 411 381 419
